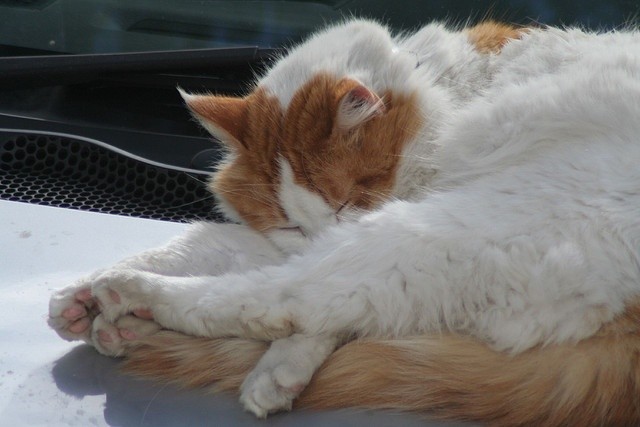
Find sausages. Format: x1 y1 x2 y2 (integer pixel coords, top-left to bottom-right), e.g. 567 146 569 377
48 15 639 425
